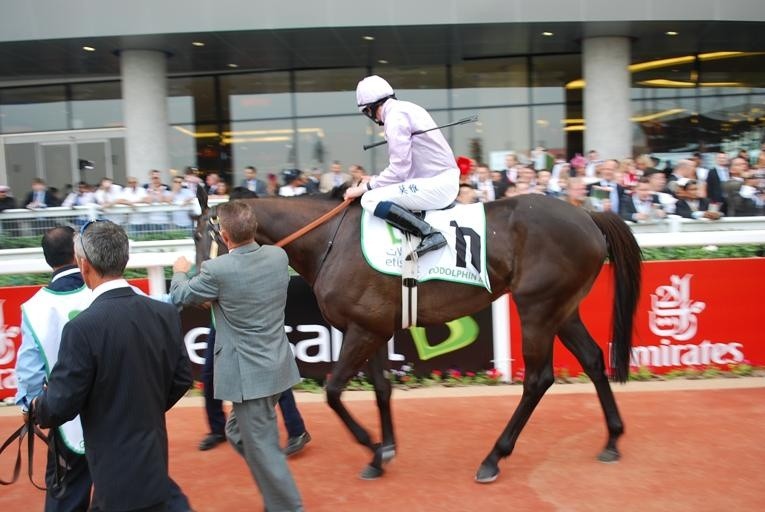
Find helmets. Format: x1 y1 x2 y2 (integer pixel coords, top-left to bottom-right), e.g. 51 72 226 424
356 75 394 112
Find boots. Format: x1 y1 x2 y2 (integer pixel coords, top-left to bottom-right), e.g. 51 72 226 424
386 204 448 261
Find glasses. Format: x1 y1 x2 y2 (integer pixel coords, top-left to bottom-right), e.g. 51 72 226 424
80 218 110 262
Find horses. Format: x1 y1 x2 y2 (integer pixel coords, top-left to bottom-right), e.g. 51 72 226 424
189 182 643 483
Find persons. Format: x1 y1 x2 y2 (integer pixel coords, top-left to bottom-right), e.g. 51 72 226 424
199 323 312 456
32 219 193 512
2 137 765 223
15 226 146 512
171 201 306 512
344 75 460 260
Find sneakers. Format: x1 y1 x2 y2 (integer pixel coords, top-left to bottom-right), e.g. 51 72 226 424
199 432 226 449
285 431 310 454
